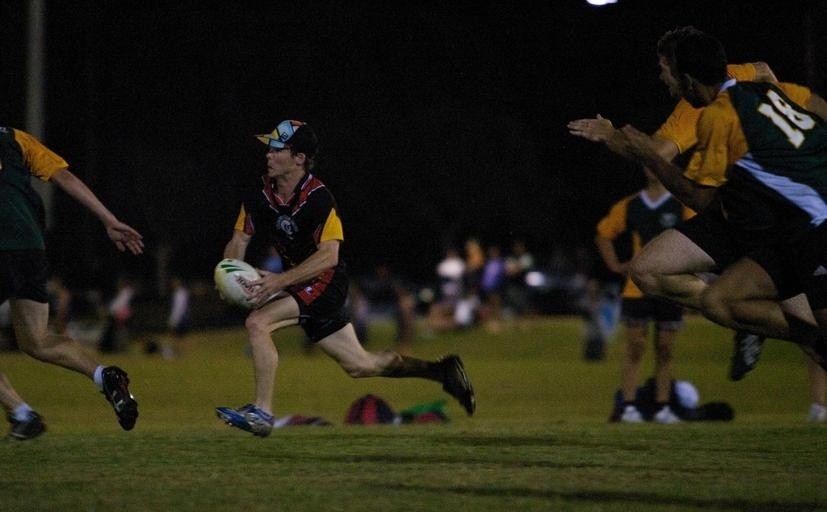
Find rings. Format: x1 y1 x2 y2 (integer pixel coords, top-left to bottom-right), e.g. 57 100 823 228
586 122 589 127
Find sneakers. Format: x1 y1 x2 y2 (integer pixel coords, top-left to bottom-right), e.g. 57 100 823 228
436 351 480 421
211 400 277 442
724 328 767 385
96 363 142 434
1 410 49 446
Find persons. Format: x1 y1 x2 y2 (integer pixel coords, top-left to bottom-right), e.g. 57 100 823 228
0 126 146 441
46 272 191 350
216 119 475 438
345 234 536 345
579 277 612 360
594 166 698 424
565 26 827 420
621 30 827 382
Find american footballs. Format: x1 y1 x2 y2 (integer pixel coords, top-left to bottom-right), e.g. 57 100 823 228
215 259 264 307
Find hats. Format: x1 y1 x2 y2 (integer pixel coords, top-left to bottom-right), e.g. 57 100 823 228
253 118 320 159
